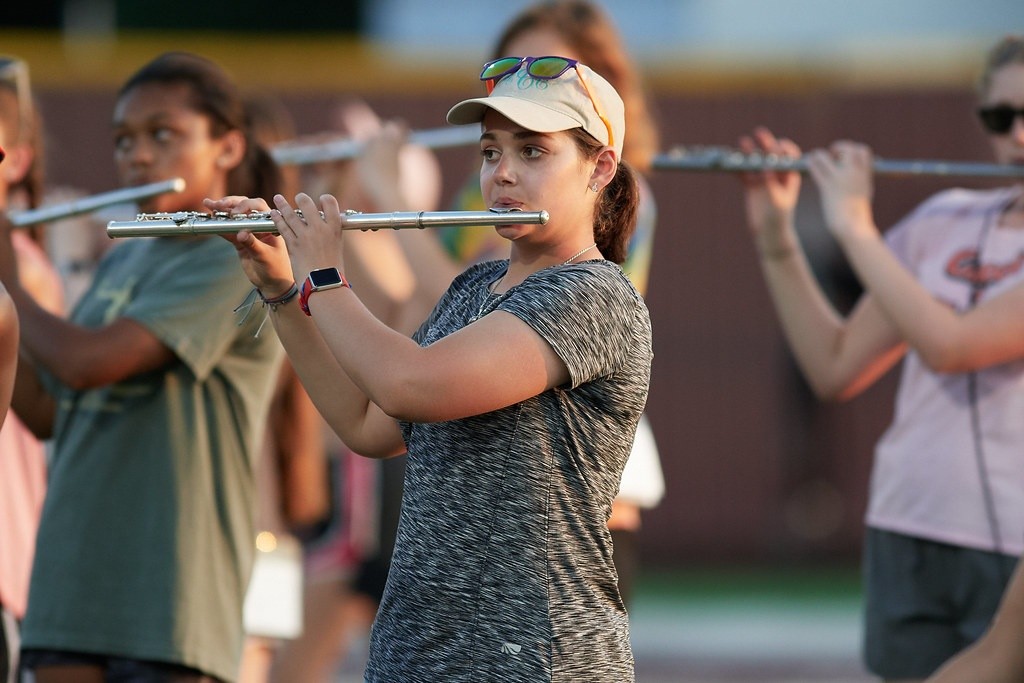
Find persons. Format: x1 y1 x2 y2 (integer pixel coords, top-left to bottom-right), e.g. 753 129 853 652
1 50 288 683
739 33 1024 683
336 0 665 617
0 145 20 432
0 56 68 617
203 56 655 683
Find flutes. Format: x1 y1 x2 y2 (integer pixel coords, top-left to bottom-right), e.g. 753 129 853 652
106 208 549 233
7 175 185 225
651 145 1023 177
265 122 480 164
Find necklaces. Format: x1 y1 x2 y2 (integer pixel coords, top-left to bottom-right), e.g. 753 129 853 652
467 242 597 324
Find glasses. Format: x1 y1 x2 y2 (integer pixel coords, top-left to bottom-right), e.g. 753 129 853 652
479 56 614 147
976 102 1023 134
1 55 31 144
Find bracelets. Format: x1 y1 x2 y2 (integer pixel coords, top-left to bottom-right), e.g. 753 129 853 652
233 280 301 338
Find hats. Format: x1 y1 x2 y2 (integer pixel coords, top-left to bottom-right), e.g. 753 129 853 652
446 61 626 165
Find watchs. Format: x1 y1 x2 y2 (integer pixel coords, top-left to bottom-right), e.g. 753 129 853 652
297 266 352 316
